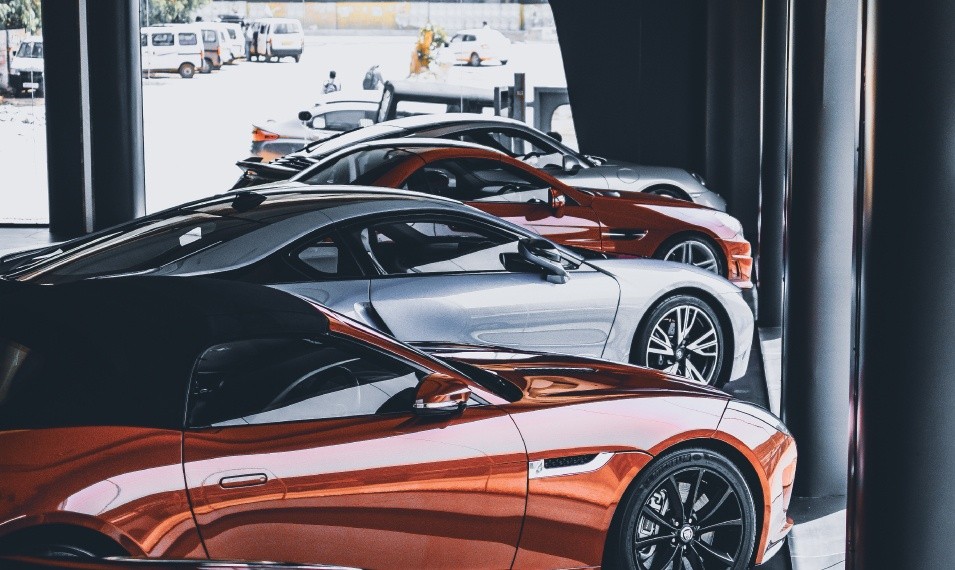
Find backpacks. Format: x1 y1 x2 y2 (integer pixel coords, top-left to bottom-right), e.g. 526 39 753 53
363 73 375 90
326 83 337 94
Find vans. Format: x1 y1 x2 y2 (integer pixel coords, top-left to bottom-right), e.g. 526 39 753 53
141 24 228 78
8 36 44 97
244 17 304 64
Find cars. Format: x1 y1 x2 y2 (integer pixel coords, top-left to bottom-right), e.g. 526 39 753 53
446 28 512 66
0 181 799 570
226 74 756 293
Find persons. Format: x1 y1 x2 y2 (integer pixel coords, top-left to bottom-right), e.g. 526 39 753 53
483 21 488 28
322 70 341 94
435 42 457 79
196 16 202 21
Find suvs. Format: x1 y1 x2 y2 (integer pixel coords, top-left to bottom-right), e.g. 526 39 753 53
196 14 251 64
